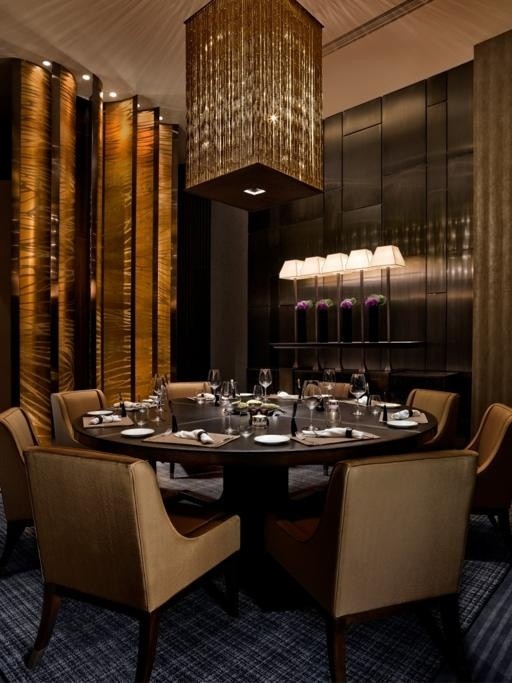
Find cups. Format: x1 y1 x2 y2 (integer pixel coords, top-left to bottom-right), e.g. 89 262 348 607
252 383 264 400
367 393 382 416
134 405 150 425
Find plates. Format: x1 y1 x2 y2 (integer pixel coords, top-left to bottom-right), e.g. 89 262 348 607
279 394 299 399
235 392 255 396
120 427 155 438
197 392 215 400
253 433 290 445
87 409 113 416
385 418 417 428
377 401 401 408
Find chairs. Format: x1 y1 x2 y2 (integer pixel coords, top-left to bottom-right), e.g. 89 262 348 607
20 445 243 681
49 382 461 443
463 401 512 547
266 450 478 681
2 403 48 579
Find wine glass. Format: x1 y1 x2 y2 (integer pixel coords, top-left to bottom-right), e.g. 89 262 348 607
322 367 336 396
348 372 366 415
299 378 323 431
207 366 222 400
151 372 167 410
258 367 272 402
219 378 242 433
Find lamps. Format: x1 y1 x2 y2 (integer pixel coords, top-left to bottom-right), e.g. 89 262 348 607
183 0 324 210
279 246 406 373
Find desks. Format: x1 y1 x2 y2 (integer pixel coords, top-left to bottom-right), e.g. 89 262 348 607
71 405 438 543
247 341 471 408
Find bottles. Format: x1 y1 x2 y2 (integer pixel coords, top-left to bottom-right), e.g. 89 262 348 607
324 399 341 427
